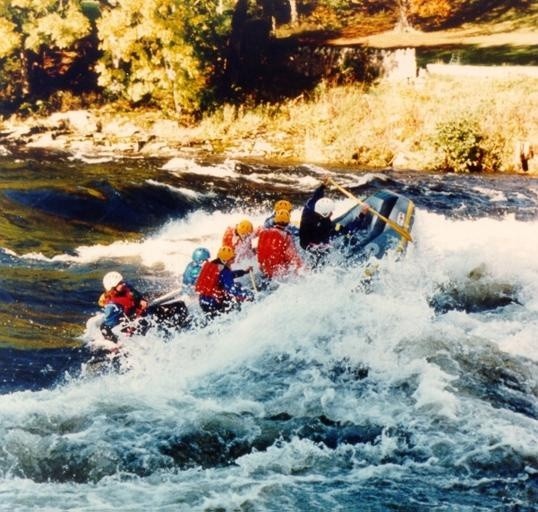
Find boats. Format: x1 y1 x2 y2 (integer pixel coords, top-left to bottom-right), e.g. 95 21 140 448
96 186 413 355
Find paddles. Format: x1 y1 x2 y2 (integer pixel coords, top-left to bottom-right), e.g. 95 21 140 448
326 179 411 243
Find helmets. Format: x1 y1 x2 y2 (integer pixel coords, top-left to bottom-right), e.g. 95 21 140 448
101 270 123 291
313 198 335 218
272 199 293 214
192 246 210 263
274 209 292 225
236 219 255 239
218 246 237 265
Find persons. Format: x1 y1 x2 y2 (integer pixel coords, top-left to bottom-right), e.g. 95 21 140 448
99 270 194 352
221 219 264 263
260 197 300 240
255 209 310 277
179 247 212 298
194 245 276 317
298 175 370 257
